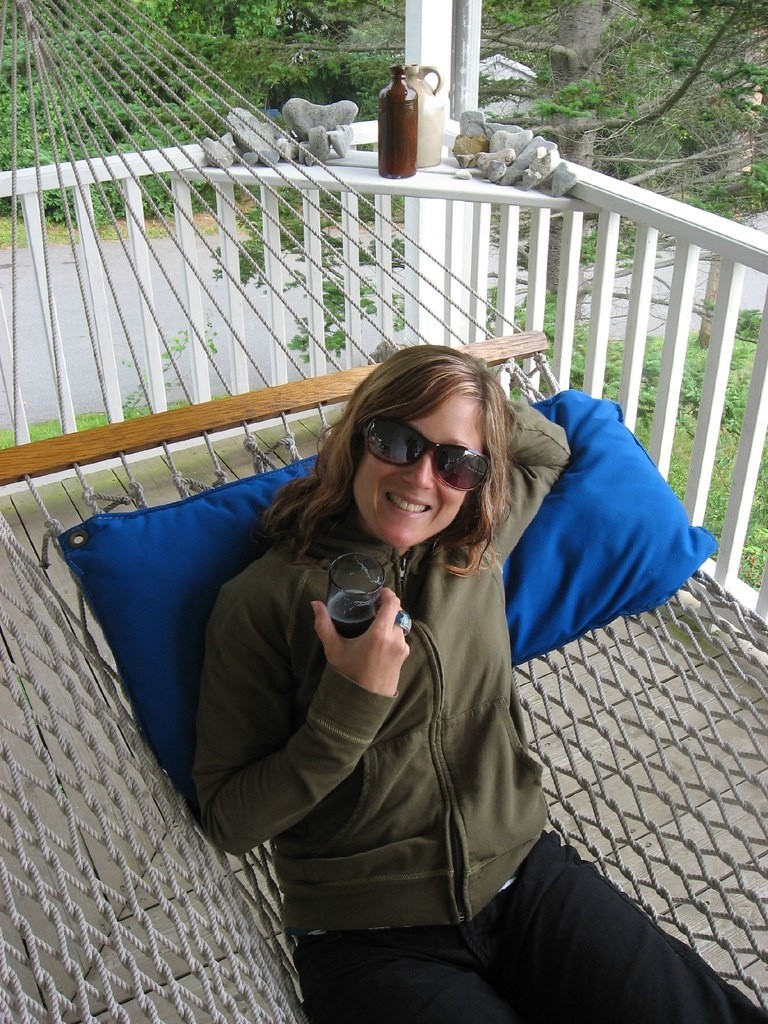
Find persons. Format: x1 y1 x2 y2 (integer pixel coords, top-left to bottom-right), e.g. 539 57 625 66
195 346 767 1023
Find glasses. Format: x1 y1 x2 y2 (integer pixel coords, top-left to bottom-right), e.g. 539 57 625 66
359 415 492 491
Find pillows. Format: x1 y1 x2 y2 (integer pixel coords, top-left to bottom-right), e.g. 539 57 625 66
54 390 721 835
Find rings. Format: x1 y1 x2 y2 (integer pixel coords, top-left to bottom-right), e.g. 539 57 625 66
395 609 412 635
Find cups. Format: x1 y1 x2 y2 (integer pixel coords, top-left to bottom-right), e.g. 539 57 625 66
326 552 386 638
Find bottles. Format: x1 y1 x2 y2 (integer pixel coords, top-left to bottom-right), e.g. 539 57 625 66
405 64 443 167
377 64 418 179
